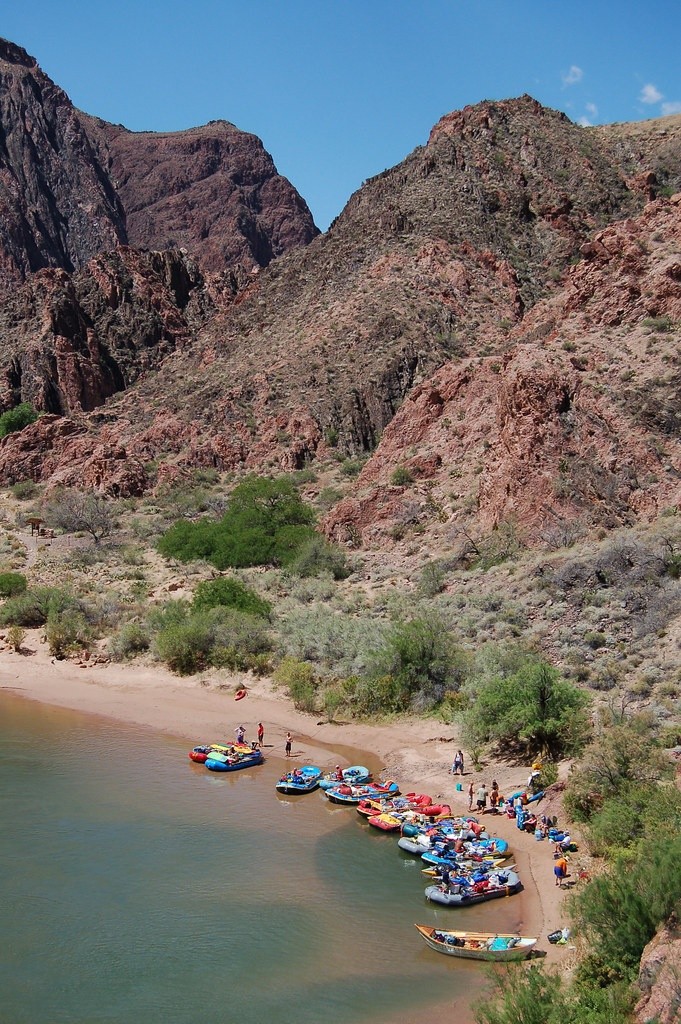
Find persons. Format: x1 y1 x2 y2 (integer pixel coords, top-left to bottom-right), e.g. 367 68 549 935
541 816 554 837
256 722 264 747
227 746 239 761
552 832 571 855
467 781 475 812
285 732 292 757
537 814 546 830
476 784 488 815
489 787 498 808
465 818 482 840
290 767 297 779
522 814 537 833
441 870 451 893
554 856 571 887
453 749 464 775
334 765 343 781
234 725 246 743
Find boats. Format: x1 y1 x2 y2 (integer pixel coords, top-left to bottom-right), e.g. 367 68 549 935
413 922 545 961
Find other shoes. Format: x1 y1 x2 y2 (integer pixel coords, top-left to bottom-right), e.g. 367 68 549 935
556 883 562 886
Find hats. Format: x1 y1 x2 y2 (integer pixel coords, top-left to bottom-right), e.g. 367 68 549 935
466 818 470 822
563 831 570 835
336 765 339 767
492 779 496 782
540 814 545 819
563 856 570 862
239 724 242 727
